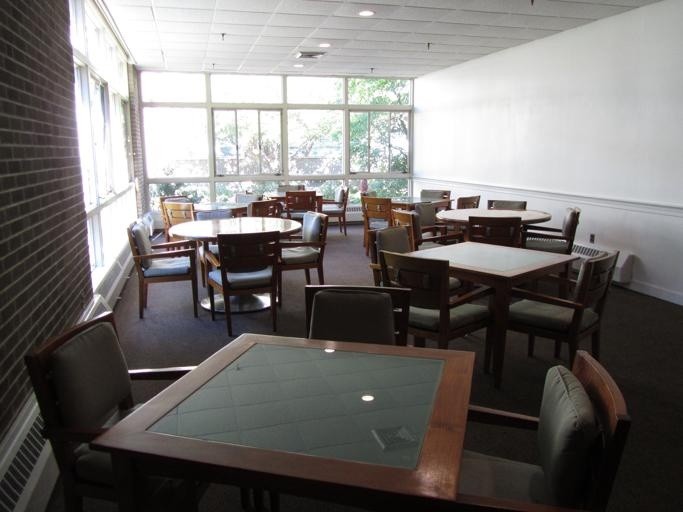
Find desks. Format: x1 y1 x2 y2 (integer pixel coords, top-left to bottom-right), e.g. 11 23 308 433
394 240 581 393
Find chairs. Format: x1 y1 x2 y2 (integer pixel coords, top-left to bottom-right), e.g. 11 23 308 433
126 185 349 338
